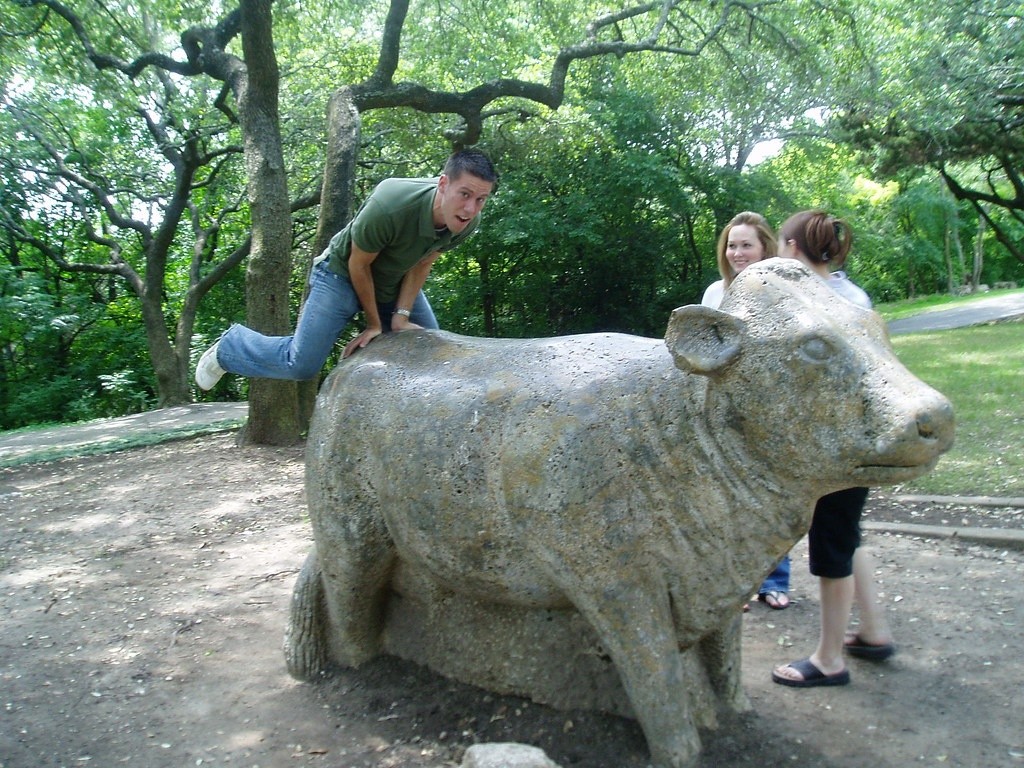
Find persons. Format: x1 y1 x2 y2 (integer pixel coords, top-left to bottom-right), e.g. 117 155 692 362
195 148 496 391
700 211 790 613
771 208 894 687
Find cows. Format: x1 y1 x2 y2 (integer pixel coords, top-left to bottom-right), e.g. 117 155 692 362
282 256 956 768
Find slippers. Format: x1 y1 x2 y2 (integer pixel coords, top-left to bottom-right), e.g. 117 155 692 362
772 657 850 688
759 591 789 609
843 632 895 660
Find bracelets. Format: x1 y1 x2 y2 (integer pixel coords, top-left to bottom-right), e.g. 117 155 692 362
391 308 411 317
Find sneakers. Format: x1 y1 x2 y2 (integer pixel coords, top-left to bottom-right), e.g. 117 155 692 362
195 339 227 391
